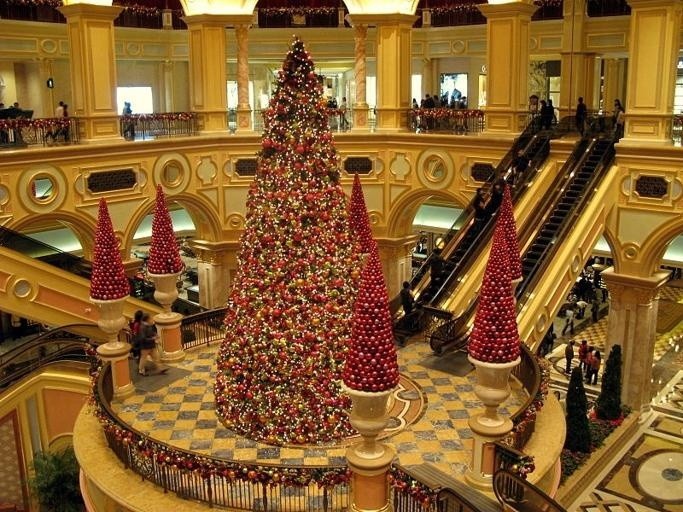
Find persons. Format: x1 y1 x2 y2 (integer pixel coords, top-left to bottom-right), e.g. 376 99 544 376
399 281 415 313
10 315 22 341
473 97 625 234
131 310 144 360
13 103 22 143
136 313 169 376
428 249 446 288
123 103 135 136
121 102 128 136
332 98 336 108
540 273 609 385
339 97 348 127
412 93 468 130
51 102 68 141
327 97 332 118
